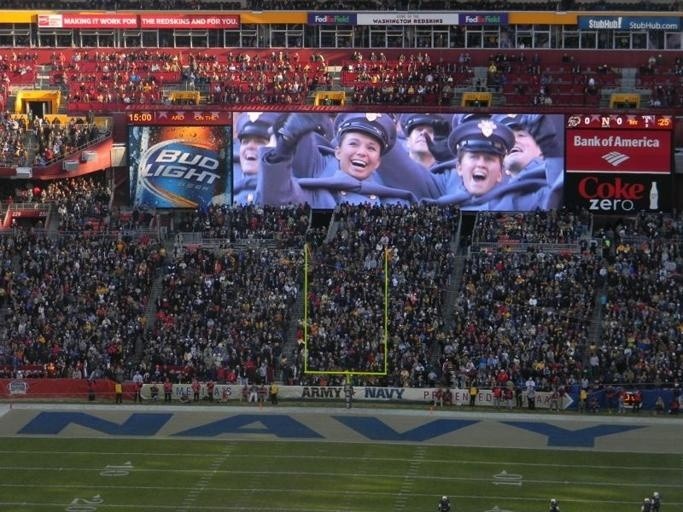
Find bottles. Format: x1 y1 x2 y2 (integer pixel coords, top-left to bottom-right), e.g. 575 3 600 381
649 181 659 210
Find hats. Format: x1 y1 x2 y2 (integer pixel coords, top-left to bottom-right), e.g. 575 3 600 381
235 111 523 157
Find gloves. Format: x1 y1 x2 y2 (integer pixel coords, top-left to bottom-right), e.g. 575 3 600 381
519 113 557 144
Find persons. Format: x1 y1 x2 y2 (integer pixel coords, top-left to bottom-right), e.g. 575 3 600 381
653 492 661 511
548 499 560 512
437 496 451 511
641 498 654 511
